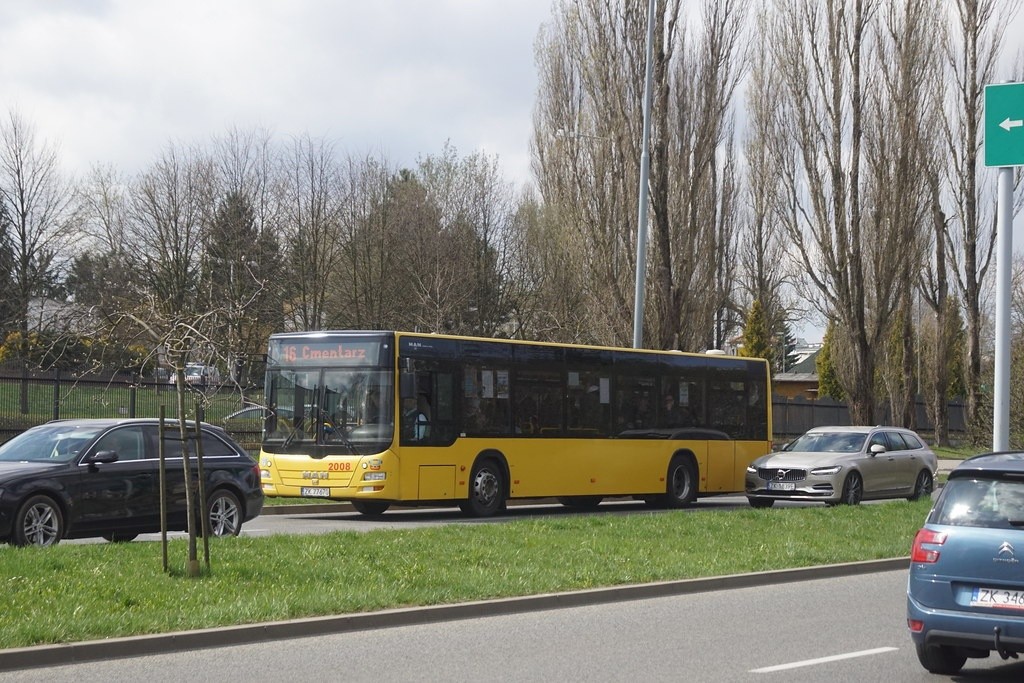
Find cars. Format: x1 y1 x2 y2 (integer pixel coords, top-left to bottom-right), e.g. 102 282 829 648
744 425 939 507
906 450 1024 675
0 417 264 551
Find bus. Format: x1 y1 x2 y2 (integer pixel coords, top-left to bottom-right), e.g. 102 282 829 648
259 331 773 519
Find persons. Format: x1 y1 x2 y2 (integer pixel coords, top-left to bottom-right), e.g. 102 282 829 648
359 389 381 423
611 389 684 432
401 395 427 444
